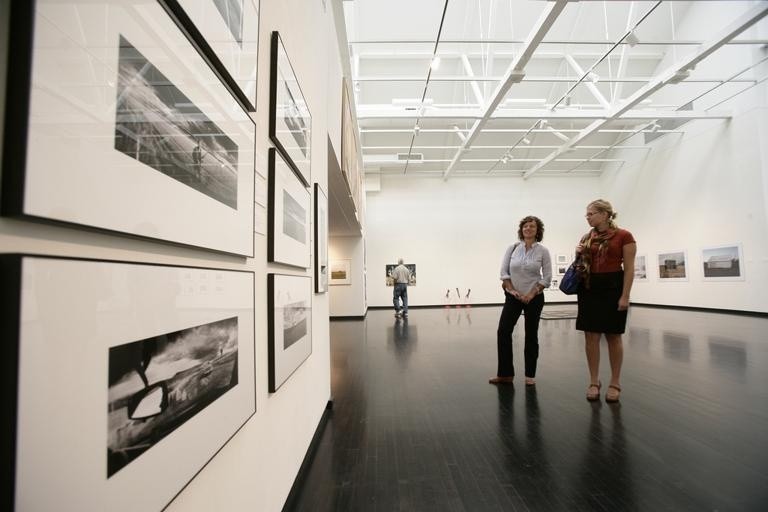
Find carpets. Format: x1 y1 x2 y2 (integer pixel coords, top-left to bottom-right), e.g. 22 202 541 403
521 307 578 320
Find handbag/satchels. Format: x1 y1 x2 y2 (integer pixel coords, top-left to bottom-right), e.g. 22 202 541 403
558 238 586 294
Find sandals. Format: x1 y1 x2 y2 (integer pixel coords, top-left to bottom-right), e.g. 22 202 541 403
586 382 600 401
606 386 620 401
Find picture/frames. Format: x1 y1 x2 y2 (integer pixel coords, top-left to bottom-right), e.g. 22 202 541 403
386 264 416 286
634 241 746 282
544 253 568 290
0 0 350 512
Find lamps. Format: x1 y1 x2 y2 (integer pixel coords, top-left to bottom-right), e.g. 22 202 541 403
414 103 425 138
501 27 662 164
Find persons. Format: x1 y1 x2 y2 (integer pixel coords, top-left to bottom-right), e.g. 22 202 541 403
575 199 637 402
489 216 553 385
392 258 410 318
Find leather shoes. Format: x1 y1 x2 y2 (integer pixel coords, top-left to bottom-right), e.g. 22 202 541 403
488 377 512 383
525 377 535 385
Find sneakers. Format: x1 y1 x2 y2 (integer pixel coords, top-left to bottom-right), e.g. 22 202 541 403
403 313 408 317
394 310 401 315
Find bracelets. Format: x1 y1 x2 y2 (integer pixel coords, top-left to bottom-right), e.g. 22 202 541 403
535 286 540 294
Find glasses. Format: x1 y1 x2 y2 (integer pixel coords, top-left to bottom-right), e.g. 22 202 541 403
584 210 599 219
